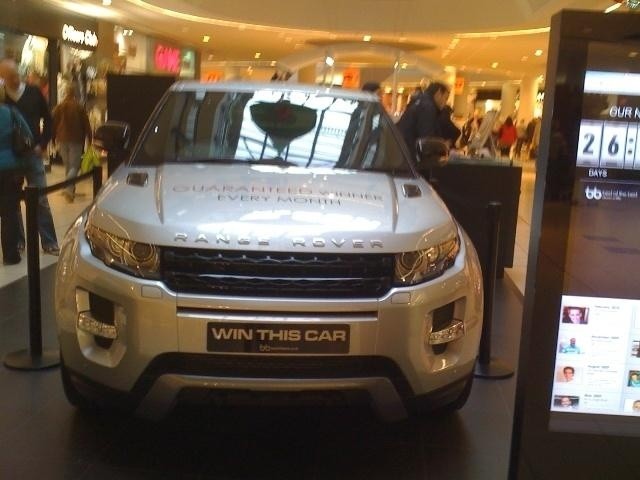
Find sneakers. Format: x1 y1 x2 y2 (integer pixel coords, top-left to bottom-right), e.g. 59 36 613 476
43 242 60 254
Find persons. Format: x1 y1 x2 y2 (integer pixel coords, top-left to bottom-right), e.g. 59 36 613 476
631 400 640 414
559 397 572 410
361 76 543 180
561 336 581 356
49 83 93 204
629 373 640 386
567 308 583 324
563 366 575 383
0 79 34 265
0 59 60 256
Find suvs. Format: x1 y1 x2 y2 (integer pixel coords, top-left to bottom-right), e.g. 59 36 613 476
53 77 486 422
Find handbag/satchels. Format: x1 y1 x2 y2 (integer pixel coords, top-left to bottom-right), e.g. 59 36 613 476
12 121 35 157
